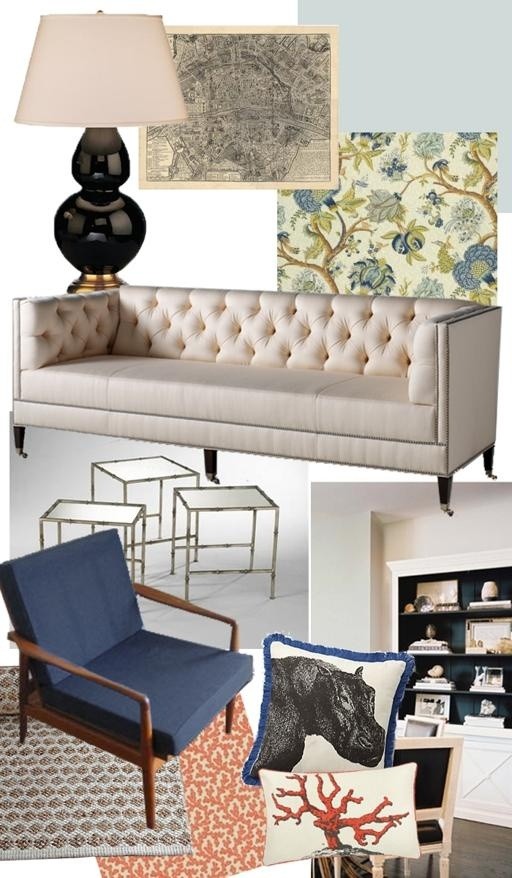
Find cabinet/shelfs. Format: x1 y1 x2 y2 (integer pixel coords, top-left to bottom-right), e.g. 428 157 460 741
386 548 512 829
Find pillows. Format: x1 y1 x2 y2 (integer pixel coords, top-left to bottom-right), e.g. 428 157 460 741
242 634 415 786
257 761 421 866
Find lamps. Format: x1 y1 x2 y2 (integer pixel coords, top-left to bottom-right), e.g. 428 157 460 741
13 9 190 298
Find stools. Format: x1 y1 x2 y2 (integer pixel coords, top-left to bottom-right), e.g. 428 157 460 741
38 455 280 604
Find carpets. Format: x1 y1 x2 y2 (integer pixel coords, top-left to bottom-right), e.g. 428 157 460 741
94 692 265 878
0 667 192 862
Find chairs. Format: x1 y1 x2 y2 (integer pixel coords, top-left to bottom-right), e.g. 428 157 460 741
0 527 255 828
333 735 464 878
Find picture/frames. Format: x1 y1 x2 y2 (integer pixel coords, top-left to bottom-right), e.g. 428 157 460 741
464 616 512 654
415 693 451 721
139 25 340 191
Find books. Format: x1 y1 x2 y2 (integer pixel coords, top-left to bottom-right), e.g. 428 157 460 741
406 639 452 654
412 676 506 693
462 714 505 728
470 601 511 609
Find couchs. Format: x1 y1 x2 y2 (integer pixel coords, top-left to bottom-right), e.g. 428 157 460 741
14 285 502 518
